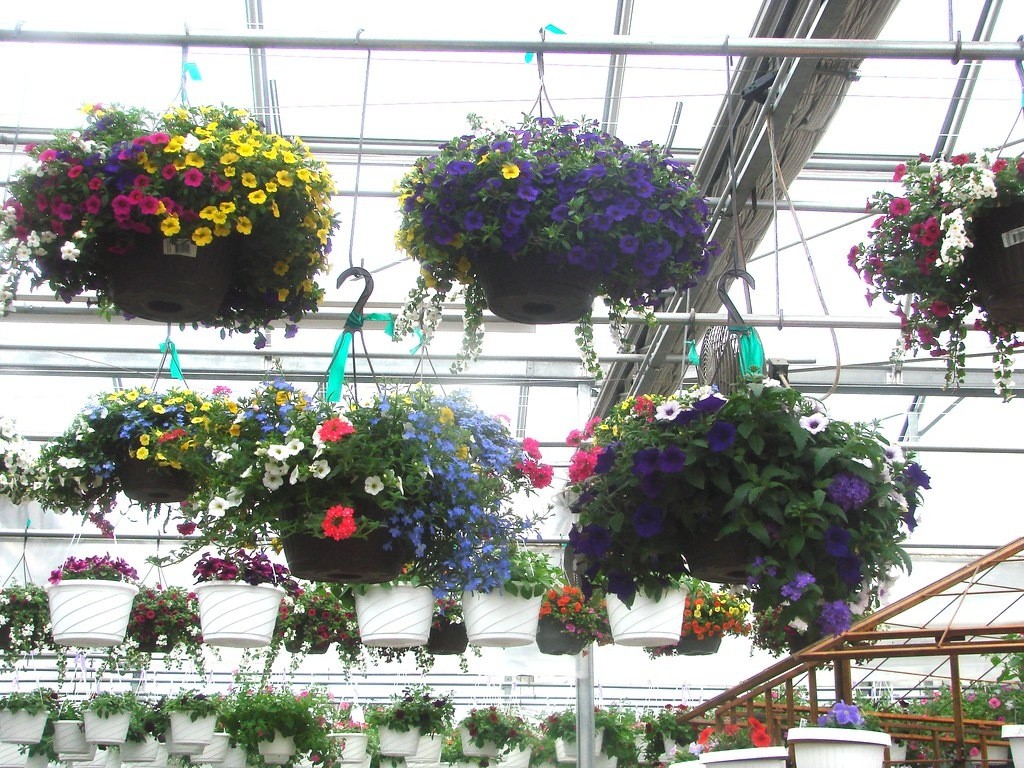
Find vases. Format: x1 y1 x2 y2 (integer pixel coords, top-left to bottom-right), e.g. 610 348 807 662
111 237 232 324
283 631 331 654
602 585 686 648
48 580 140 647
112 457 196 504
955 204 1024 328
282 528 412 584
2 711 906 766
681 633 724 654
971 746 1009 765
478 244 596 322
135 629 175 653
194 581 287 646
1000 724 1024 767
364 581 433 649
425 613 468 655
461 588 539 646
689 522 762 582
0 625 10 648
539 617 587 657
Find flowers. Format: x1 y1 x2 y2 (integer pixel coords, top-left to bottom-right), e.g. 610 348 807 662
815 701 866 731
390 118 716 388
1 357 770 767
0 97 332 345
867 632 1024 768
846 151 1024 403
563 373 933 641
